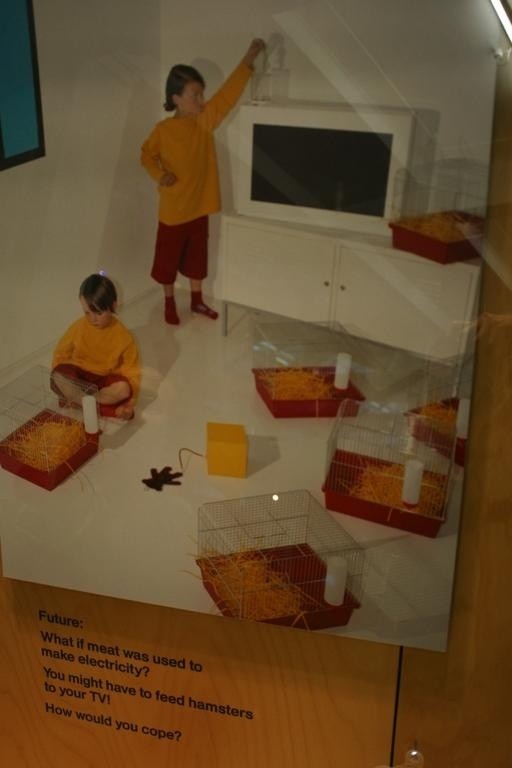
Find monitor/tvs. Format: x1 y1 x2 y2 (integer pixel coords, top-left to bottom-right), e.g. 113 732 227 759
231 100 414 236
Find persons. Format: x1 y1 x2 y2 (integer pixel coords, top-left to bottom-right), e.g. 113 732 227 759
139 37 264 324
48 272 141 422
